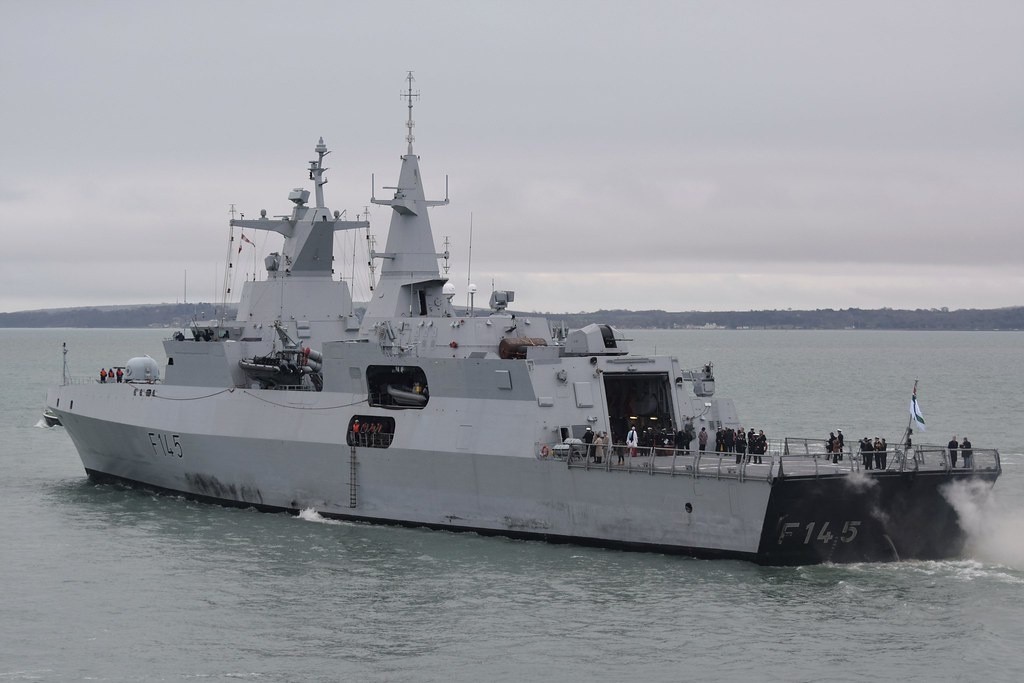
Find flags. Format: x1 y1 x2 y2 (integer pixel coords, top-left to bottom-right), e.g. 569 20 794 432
910 382 925 432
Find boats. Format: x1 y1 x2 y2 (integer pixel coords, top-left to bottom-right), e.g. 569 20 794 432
385 379 428 408
238 357 313 387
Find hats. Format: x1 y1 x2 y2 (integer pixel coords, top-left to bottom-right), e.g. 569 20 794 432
648 427 652 430
717 427 722 429
586 428 591 430
631 427 636 430
837 429 842 432
753 434 759 438
875 436 879 440
643 431 647 433
750 427 754 429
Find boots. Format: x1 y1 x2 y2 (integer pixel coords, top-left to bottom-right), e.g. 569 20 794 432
599 458 601 463
592 457 595 463
596 458 599 463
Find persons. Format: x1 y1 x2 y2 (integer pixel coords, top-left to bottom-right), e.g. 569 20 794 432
676 418 695 455
413 382 428 402
860 437 887 469
947 436 958 468
100 368 123 383
618 440 626 464
352 420 383 443
715 427 766 463
582 427 609 463
698 427 708 455
640 427 669 456
960 437 971 467
627 426 637 455
826 429 844 463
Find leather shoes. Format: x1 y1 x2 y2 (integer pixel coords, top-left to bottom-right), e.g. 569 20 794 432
875 467 879 469
865 468 868 470
869 468 874 470
879 468 885 470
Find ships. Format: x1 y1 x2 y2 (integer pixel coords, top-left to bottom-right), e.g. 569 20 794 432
42 72 1004 572
